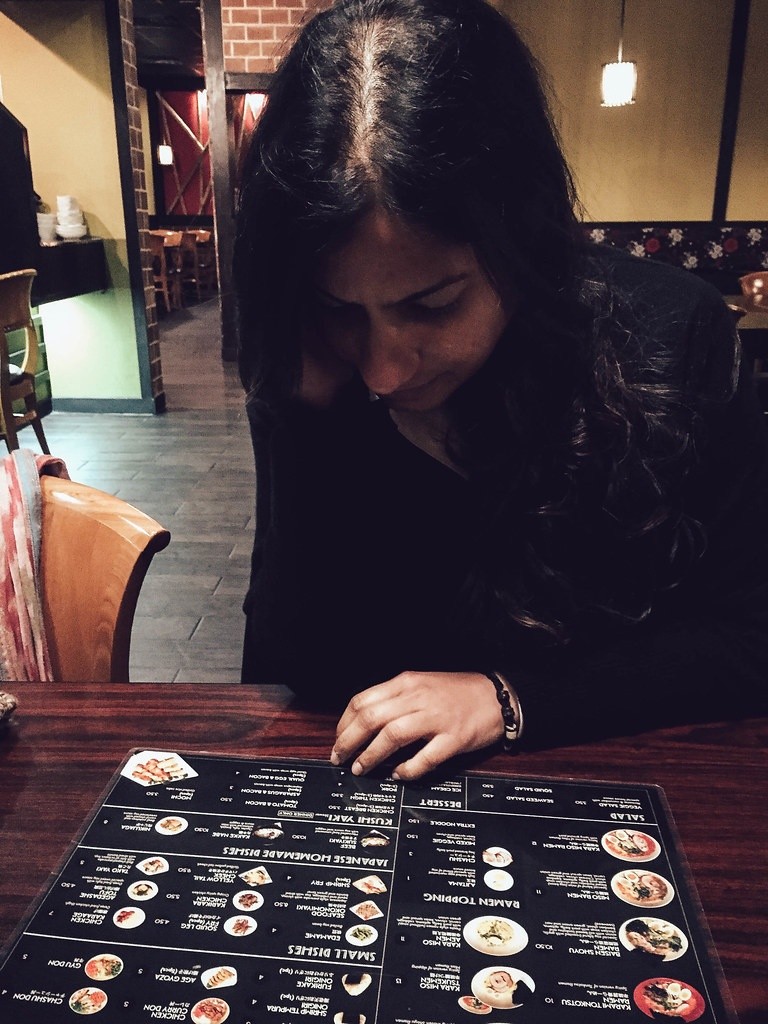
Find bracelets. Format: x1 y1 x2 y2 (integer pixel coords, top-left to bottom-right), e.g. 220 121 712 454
487 672 518 752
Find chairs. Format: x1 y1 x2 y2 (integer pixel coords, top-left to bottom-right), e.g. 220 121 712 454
0 457 171 685
0 266 55 458
150 224 215 312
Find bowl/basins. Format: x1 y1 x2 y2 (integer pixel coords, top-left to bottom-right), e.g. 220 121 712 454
37 195 87 241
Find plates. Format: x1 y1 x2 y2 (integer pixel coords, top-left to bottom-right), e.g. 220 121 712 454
352 875 387 894
611 869 675 908
458 966 535 1015
127 880 158 901
120 751 198 787
191 966 238 1024
155 816 188 836
634 978 705 1023
484 847 514 891
112 907 146 929
601 829 661 863
224 865 272 937
136 857 169 875
85 954 124 981
345 924 378 947
69 987 108 1015
463 915 529 956
350 900 384 921
619 917 688 961
251 826 284 845
358 834 390 854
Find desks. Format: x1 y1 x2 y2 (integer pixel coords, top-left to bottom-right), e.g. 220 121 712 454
0 685 768 1024
722 293 768 416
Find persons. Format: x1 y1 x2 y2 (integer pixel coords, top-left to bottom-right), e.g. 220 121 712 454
230 0 768 782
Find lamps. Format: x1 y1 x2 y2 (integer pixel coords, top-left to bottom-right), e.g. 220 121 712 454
156 128 174 167
602 0 644 108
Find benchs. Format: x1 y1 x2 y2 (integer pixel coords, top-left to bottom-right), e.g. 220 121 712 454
578 221 768 294
